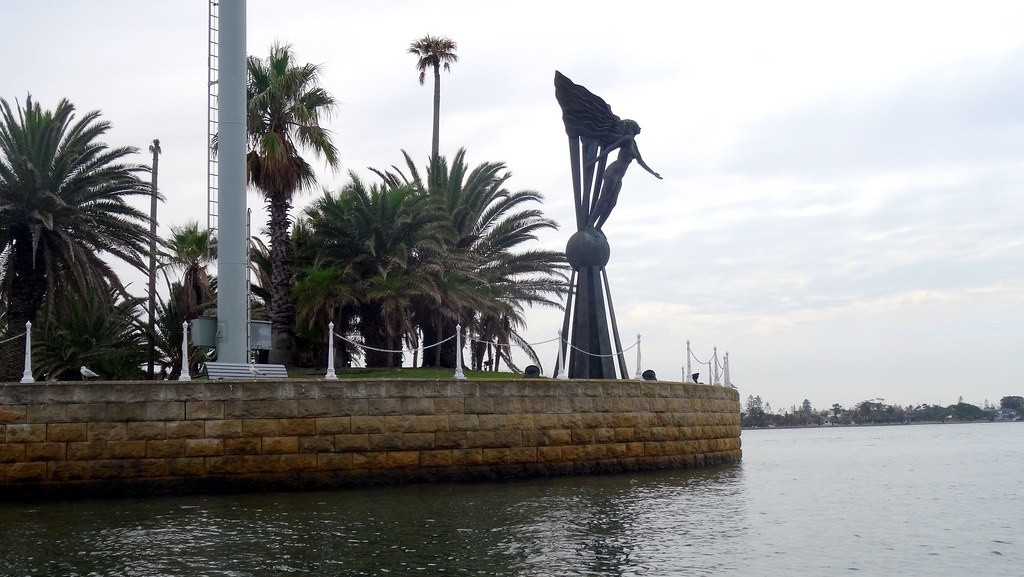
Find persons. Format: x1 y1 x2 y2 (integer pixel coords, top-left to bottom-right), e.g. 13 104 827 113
554 70 664 239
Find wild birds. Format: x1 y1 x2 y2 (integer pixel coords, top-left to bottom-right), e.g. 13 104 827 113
80 366 99 382
